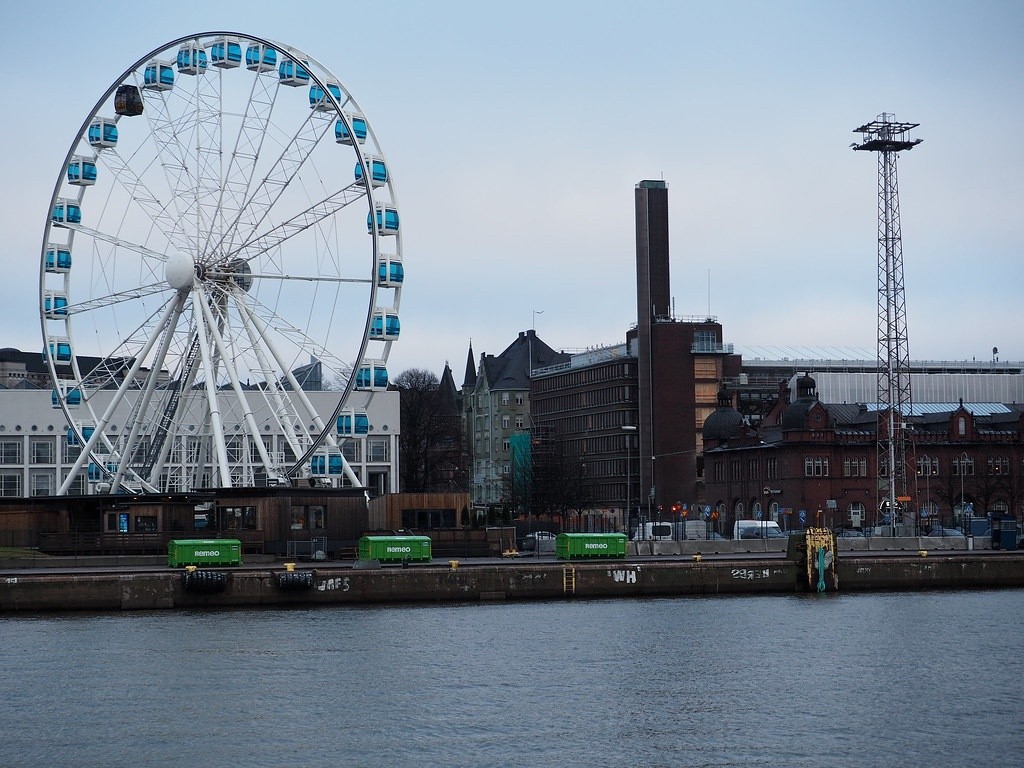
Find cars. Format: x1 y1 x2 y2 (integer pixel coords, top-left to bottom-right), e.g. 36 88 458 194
527 531 557 540
741 526 786 538
928 529 964 536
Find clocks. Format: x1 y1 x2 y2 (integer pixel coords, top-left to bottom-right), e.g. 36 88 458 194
814 412 823 422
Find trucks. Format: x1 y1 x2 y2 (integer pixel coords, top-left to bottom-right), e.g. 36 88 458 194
632 521 673 541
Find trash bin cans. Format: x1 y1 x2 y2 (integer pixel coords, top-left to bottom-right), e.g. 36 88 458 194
356 535 432 561
166 538 244 566
555 532 628 557
517 535 536 557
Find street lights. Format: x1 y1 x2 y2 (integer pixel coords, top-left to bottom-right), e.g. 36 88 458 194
961 452 970 534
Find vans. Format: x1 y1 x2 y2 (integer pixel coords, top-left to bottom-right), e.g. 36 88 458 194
733 520 781 539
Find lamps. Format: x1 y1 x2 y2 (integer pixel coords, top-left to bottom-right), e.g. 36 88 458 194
865 489 869 494
937 488 940 493
844 490 848 494
918 489 921 493
